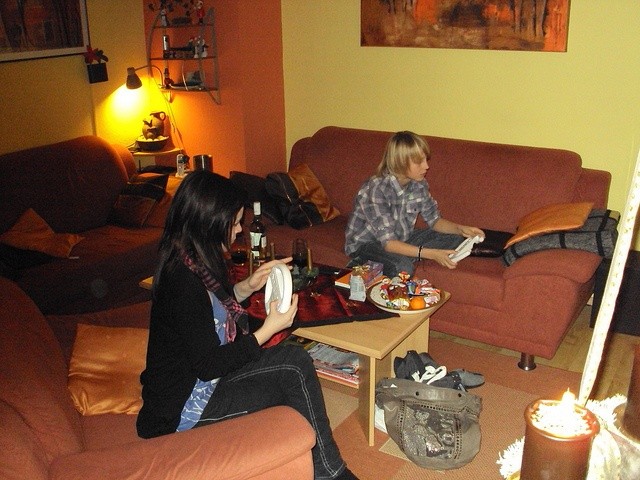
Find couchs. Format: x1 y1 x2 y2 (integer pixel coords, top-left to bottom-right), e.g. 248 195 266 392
0 135 182 314
240 122 612 371
0 275 317 480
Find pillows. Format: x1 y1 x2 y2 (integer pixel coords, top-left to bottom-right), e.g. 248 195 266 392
113 165 176 230
65 320 150 417
470 227 514 257
264 163 343 229
229 169 284 226
2 206 85 260
505 199 594 249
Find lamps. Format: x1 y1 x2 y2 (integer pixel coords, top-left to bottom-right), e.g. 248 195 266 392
125 65 163 90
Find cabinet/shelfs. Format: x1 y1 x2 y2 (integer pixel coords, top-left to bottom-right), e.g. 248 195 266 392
147 5 222 106
127 147 185 172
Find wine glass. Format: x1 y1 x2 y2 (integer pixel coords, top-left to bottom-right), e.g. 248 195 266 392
292 238 308 278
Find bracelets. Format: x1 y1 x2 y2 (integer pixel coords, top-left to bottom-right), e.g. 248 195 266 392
417 245 423 261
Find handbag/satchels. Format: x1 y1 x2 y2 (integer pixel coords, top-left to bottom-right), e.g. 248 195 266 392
375 377 481 469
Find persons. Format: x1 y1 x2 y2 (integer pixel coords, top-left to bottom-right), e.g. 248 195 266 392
344 131 486 284
136 170 362 480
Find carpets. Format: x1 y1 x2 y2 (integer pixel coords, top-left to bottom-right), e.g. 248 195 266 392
329 337 582 480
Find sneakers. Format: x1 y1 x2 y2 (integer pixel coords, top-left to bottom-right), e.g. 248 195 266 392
448 368 485 387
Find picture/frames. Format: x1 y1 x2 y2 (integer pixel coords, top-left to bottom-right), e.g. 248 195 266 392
0 1 91 63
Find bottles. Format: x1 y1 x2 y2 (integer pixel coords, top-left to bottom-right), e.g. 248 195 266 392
231 218 247 267
249 201 267 268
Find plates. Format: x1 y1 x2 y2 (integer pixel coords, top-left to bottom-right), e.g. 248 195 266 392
366 281 445 313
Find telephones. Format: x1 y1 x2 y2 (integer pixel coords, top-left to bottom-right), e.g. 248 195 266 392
175 153 188 179
